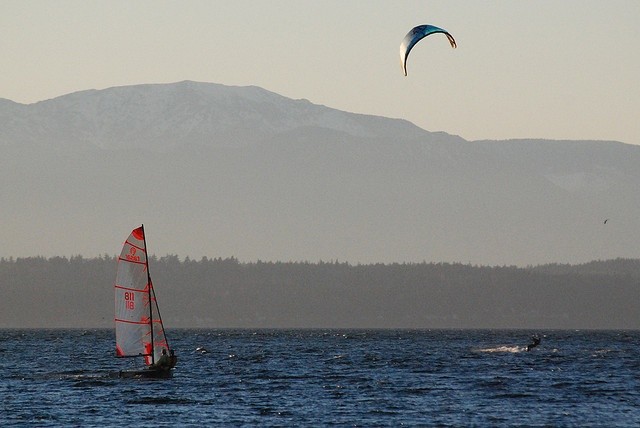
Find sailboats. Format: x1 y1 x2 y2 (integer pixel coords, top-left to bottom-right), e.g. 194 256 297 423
115 224 178 371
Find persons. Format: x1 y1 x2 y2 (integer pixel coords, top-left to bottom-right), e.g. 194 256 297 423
157 349 168 366
166 349 177 368
528 337 542 351
